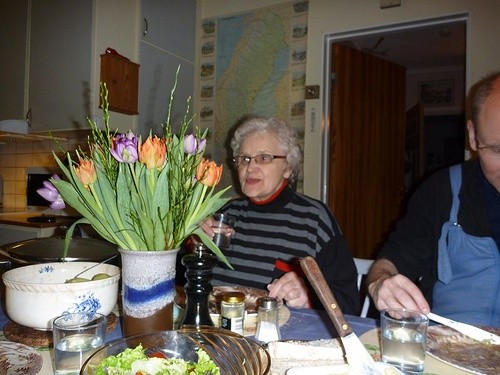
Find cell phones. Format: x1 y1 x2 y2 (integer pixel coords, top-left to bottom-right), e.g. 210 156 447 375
27 216 56 223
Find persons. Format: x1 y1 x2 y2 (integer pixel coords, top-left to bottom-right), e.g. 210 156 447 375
363 70 500 329
179 113 361 316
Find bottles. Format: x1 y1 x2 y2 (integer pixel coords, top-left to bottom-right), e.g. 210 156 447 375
181 242 214 328
256 297 281 345
220 292 246 337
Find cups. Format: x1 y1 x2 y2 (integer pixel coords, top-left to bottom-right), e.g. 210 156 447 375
52 312 107 375
379 308 429 375
208 212 235 249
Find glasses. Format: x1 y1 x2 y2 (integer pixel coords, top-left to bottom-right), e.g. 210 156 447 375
474 138 500 155
233 154 286 166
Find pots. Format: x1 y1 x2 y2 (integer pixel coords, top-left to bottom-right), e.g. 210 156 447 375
1 227 120 273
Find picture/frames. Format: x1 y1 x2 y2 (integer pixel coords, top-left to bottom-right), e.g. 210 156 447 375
417 79 454 105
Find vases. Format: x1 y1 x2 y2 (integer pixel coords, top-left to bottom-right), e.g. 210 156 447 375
117 247 181 319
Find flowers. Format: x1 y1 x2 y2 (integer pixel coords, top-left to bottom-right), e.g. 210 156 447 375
37 63 235 261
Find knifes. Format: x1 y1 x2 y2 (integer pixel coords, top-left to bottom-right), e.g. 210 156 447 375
427 311 500 346
301 257 383 374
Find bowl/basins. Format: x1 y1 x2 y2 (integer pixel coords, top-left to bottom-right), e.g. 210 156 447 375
2 261 121 331
78 325 271 375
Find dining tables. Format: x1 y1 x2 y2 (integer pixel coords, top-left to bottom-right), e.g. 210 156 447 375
106 302 382 346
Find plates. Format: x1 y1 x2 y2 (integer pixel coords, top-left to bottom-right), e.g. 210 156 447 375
423 323 500 375
287 361 405 375
173 284 285 316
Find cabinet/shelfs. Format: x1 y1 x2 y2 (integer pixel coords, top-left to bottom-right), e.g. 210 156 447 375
0 0 200 134
